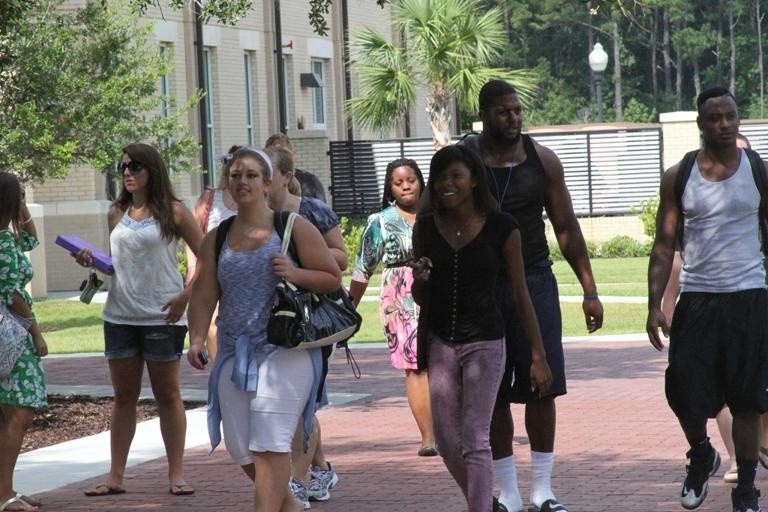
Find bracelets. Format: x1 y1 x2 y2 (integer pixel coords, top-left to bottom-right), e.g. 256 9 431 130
18 217 32 225
584 295 598 299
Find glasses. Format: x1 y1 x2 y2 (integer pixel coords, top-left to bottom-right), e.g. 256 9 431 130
117 161 143 174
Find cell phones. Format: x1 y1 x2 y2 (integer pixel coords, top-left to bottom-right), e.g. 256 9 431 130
198 352 208 365
417 257 432 278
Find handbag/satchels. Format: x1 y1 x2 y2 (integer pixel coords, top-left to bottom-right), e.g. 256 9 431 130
0 300 31 380
267 278 361 350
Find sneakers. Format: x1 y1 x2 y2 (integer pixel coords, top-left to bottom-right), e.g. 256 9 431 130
723 445 768 512
287 462 338 510
418 447 437 455
679 439 720 509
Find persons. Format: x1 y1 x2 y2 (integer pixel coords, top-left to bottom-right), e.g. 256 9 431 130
185 134 349 511
407 145 553 512
663 134 768 483
419 81 604 512
645 86 768 512
71 144 204 496
1 172 49 511
349 160 441 455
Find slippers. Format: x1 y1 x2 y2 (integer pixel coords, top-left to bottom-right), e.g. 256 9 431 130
527 499 568 512
169 485 193 495
0 493 31 512
84 484 125 496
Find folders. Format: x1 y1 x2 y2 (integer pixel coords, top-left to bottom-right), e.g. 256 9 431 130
54 235 113 273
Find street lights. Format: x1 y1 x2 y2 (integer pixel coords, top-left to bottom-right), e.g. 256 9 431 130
588 42 608 124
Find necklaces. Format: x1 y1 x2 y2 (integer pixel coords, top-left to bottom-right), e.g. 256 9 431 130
481 144 515 205
441 210 476 237
